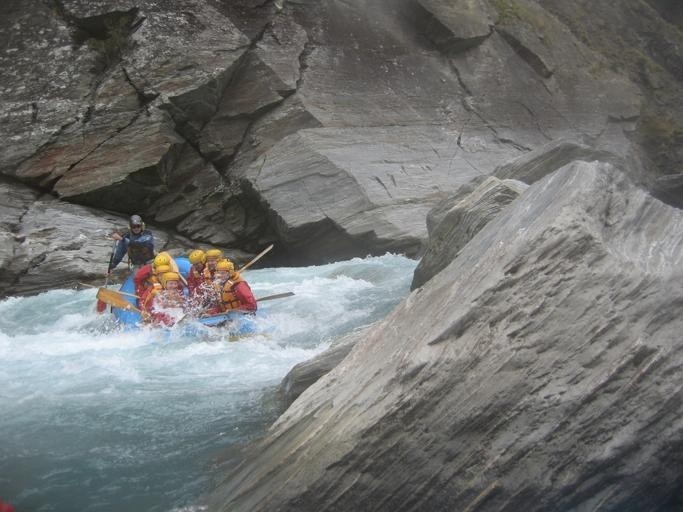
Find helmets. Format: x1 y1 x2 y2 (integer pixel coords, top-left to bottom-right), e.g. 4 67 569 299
129 214 142 228
189 250 234 274
154 254 180 286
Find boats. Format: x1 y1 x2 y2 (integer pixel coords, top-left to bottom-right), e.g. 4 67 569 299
111 257 280 344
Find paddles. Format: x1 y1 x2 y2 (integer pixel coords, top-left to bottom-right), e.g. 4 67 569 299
237 244 273 275
255 291 295 301
97 239 117 311
95 288 152 320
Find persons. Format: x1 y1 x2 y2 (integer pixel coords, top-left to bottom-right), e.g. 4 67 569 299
132 247 256 325
104 215 156 278
